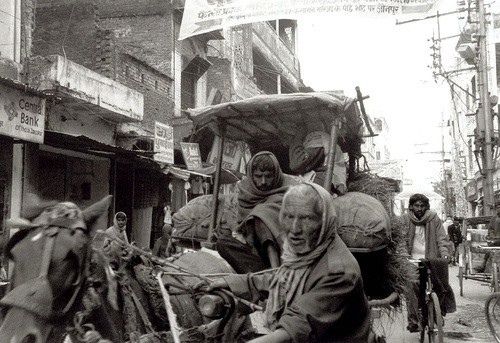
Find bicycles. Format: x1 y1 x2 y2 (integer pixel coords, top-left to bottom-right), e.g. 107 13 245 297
406 256 454 343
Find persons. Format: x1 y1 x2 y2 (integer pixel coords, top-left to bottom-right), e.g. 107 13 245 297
472 200 500 273
152 223 176 258
101 211 135 252
442 216 453 234
236 149 298 272
398 194 452 332
448 218 463 266
192 184 375 342
278 119 348 188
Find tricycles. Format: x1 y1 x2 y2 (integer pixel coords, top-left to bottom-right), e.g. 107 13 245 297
458 216 500 293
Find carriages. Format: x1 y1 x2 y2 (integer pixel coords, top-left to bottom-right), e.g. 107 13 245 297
0 85 420 343
